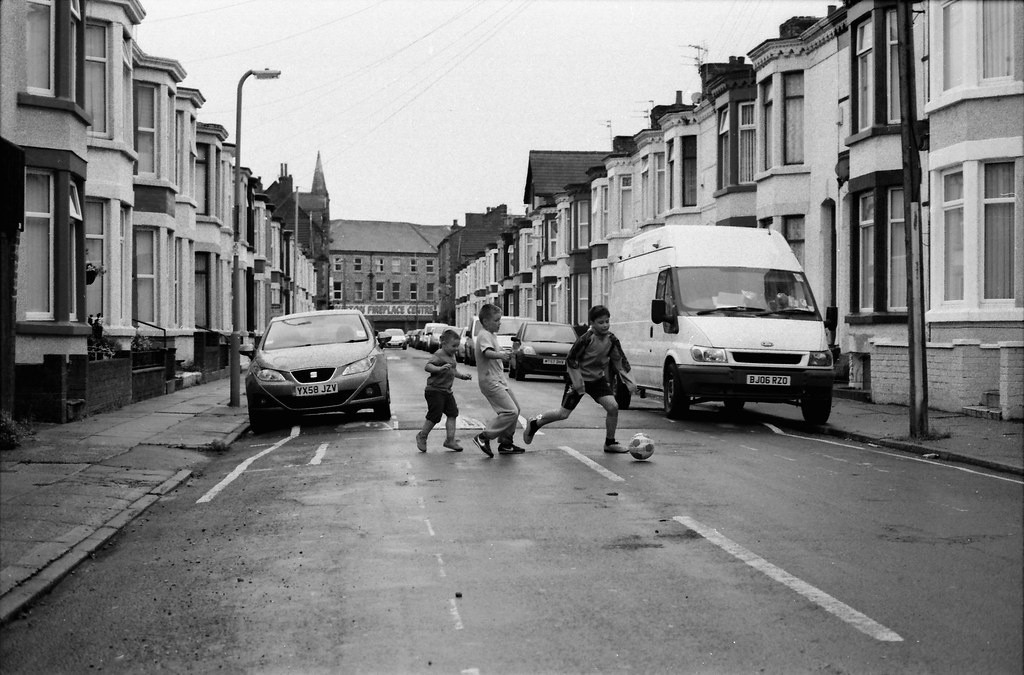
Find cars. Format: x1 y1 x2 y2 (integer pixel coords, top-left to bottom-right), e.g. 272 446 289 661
383 328 407 350
465 316 536 368
407 322 469 363
237 308 392 435
508 322 580 384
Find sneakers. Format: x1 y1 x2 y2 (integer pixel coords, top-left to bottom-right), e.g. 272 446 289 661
443 439 463 451
472 434 494 458
416 432 427 452
498 444 525 454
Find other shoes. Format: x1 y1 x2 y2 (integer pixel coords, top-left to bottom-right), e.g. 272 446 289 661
523 417 537 444
604 441 629 453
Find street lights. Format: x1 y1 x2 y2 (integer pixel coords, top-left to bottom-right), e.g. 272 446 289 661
228 68 282 405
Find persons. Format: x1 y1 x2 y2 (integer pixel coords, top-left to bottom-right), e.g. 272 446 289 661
472 304 526 458
415 329 472 455
523 305 631 458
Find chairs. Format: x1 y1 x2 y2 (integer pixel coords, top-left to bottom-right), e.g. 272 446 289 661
332 326 356 342
525 329 567 341
279 330 305 343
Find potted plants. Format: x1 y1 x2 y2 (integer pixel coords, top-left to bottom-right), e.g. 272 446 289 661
131 334 163 369
86 247 106 285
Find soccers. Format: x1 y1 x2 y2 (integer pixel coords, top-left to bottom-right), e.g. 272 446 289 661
628 433 655 460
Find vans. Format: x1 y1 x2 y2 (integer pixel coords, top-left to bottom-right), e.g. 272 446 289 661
608 225 839 426
